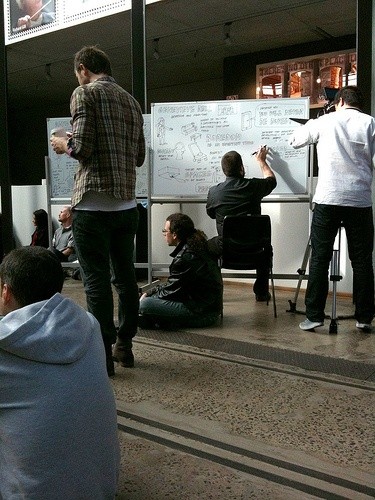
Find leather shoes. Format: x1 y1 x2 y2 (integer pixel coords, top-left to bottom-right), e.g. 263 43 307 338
255 292 271 302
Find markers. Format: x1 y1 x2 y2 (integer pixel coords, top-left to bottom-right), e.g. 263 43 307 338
252 145 267 156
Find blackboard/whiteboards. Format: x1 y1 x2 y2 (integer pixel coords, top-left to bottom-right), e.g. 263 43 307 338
45 113 151 205
150 96 313 205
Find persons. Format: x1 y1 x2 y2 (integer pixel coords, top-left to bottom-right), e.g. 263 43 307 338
288 86 375 330
138 212 223 331
205 146 277 301
0 206 78 264
49 46 146 376
0 246 122 500
12 0 54 33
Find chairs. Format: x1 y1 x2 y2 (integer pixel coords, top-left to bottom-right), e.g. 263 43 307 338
218 216 277 318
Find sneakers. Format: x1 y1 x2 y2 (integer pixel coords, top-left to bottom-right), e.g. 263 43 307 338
356 321 372 330
299 318 324 329
112 349 134 368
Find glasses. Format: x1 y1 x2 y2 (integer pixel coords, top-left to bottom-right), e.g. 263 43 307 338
161 228 171 233
